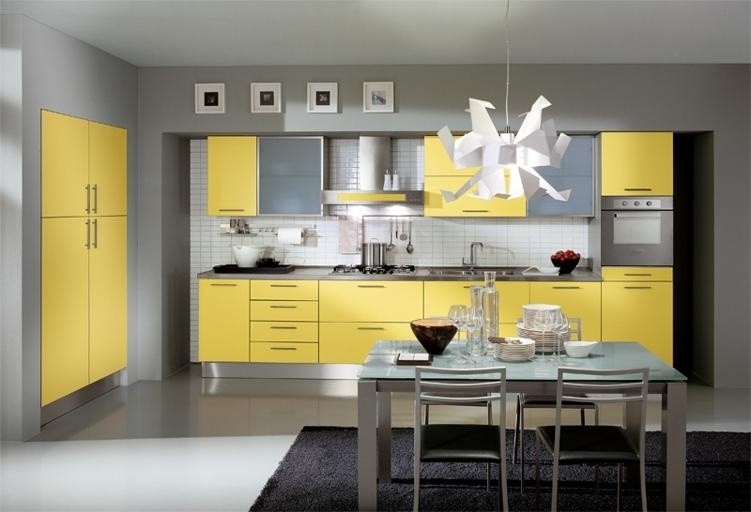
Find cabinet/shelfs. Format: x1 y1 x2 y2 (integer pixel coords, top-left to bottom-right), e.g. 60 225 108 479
258 135 329 218
424 281 529 344
200 280 249 364
319 281 424 364
601 266 673 366
596 131 673 197
249 280 320 363
208 136 257 215
529 284 601 342
527 134 595 217
423 136 528 219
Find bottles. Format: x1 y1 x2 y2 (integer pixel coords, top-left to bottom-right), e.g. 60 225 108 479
467 288 485 355
483 270 502 356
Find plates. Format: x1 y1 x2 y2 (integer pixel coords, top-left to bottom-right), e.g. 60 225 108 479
494 337 536 362
518 305 571 354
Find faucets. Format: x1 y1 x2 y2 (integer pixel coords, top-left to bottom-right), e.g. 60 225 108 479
469 242 483 264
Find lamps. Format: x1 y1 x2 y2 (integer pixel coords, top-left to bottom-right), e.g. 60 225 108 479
436 2 571 205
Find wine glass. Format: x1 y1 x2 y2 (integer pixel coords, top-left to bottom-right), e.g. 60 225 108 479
449 304 483 356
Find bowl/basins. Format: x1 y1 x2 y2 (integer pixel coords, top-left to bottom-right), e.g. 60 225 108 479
562 339 597 359
409 320 458 355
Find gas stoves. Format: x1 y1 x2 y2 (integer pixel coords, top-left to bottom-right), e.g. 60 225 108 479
329 264 417 276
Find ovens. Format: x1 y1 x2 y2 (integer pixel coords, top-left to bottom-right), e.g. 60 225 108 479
588 196 676 267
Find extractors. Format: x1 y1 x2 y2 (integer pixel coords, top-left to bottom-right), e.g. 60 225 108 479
320 136 423 206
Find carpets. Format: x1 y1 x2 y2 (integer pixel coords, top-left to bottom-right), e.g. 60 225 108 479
250 424 751 511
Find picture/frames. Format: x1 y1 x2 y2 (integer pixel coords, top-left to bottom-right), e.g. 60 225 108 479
362 81 395 113
195 82 226 115
251 83 281 113
307 82 339 113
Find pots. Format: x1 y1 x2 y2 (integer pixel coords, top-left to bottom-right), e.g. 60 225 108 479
363 238 391 267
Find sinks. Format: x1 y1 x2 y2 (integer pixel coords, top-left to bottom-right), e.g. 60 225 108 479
475 270 515 276
429 269 473 277
